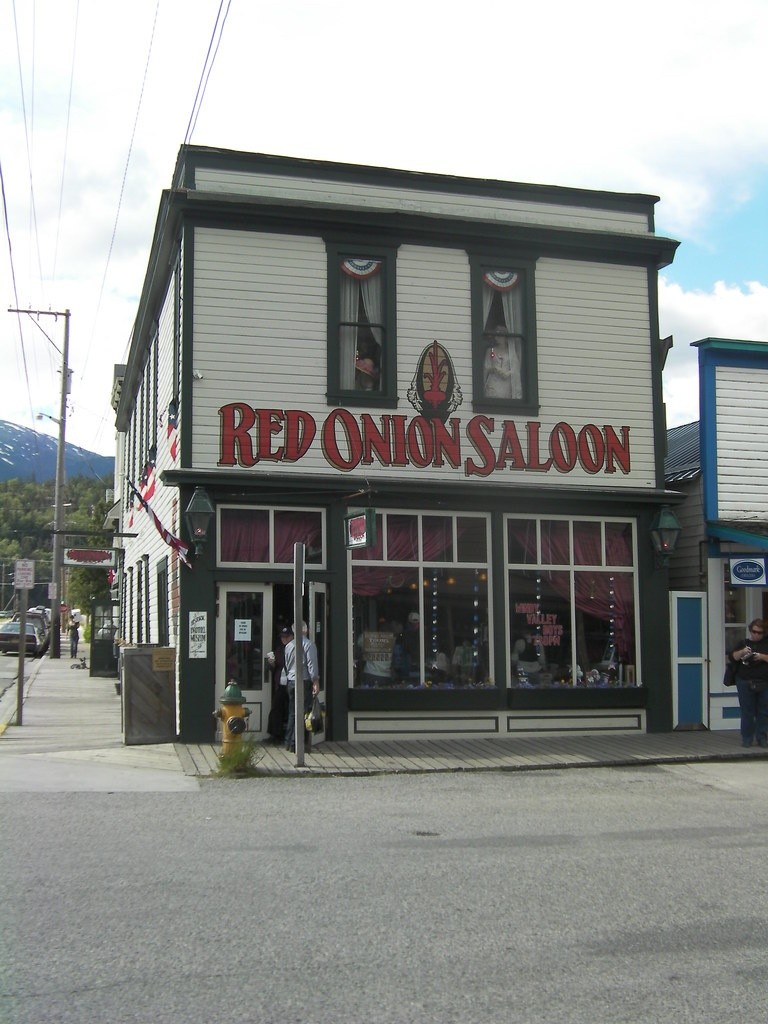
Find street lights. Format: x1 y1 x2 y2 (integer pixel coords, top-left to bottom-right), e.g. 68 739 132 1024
35 412 66 659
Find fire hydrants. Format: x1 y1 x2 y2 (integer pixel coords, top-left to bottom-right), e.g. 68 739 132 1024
213 680 254 770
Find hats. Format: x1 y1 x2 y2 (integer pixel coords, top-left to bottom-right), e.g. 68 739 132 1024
279 627 292 636
407 612 419 623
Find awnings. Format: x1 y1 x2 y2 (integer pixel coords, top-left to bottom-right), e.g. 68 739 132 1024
706 524 768 549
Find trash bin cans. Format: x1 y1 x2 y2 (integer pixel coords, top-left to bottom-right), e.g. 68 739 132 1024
118 646 178 745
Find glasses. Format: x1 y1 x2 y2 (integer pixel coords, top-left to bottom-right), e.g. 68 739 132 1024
751 629 764 634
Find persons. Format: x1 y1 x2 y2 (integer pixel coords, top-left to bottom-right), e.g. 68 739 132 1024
248 626 279 690
264 619 320 753
354 604 472 680
728 618 768 749
486 327 511 398
66 615 80 658
511 628 541 674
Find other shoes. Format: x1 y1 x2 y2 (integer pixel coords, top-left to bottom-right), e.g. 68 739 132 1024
743 743 750 747
758 741 767 747
286 742 295 752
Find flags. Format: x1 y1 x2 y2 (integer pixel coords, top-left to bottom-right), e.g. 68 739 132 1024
135 445 157 512
129 486 134 528
166 401 179 463
126 476 193 571
108 569 116 583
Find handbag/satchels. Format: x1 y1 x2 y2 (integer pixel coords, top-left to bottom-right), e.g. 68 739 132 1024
307 694 325 746
304 694 325 731
723 639 749 686
279 667 287 685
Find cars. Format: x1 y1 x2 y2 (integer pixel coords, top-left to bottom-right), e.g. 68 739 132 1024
0 622 43 658
10 605 53 634
